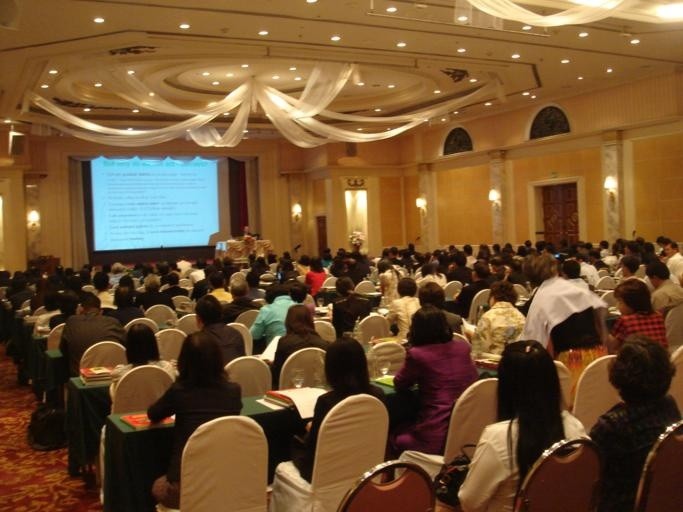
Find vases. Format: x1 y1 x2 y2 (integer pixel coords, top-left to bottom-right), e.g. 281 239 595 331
352 245 361 257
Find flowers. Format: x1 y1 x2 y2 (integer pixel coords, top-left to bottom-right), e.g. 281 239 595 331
347 231 366 246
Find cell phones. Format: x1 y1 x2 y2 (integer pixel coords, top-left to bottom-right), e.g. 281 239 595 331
552 251 562 259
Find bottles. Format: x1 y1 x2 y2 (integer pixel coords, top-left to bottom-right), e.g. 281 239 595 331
476 306 484 324
365 335 379 380
277 268 283 281
470 328 482 358
353 316 363 344
524 281 533 297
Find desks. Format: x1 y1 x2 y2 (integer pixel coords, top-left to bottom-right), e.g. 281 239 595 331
214 239 275 264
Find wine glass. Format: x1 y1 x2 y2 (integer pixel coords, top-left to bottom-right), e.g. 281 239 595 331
317 298 324 311
290 368 304 389
378 359 391 382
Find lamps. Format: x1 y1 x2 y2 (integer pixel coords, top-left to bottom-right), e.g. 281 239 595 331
603 175 618 189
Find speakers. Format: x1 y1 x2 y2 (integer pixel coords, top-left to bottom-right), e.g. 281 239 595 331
7 130 27 156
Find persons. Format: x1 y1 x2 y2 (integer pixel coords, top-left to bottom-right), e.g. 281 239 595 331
2 227 683 512
